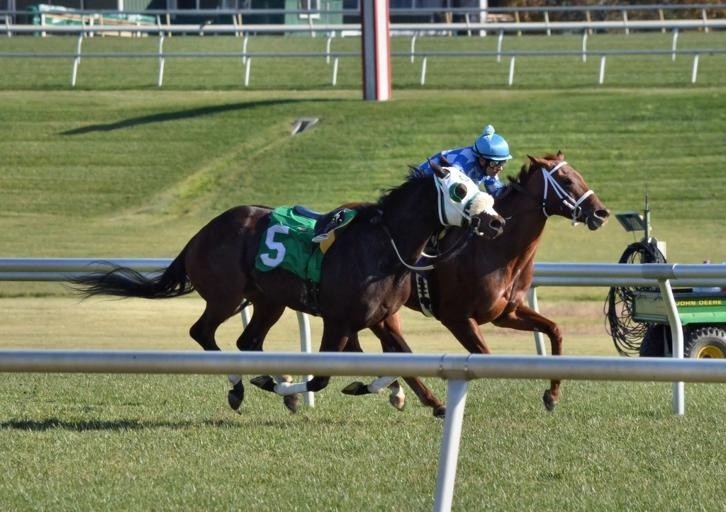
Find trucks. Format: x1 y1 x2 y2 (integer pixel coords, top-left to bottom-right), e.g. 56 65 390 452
616 189 726 360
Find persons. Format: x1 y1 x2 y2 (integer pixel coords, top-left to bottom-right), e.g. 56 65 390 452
410 124 514 199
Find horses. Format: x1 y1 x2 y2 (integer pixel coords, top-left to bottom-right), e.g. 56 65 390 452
61 153 506 413
232 150 611 418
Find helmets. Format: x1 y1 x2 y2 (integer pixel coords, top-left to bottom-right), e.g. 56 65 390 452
472 125 513 161
